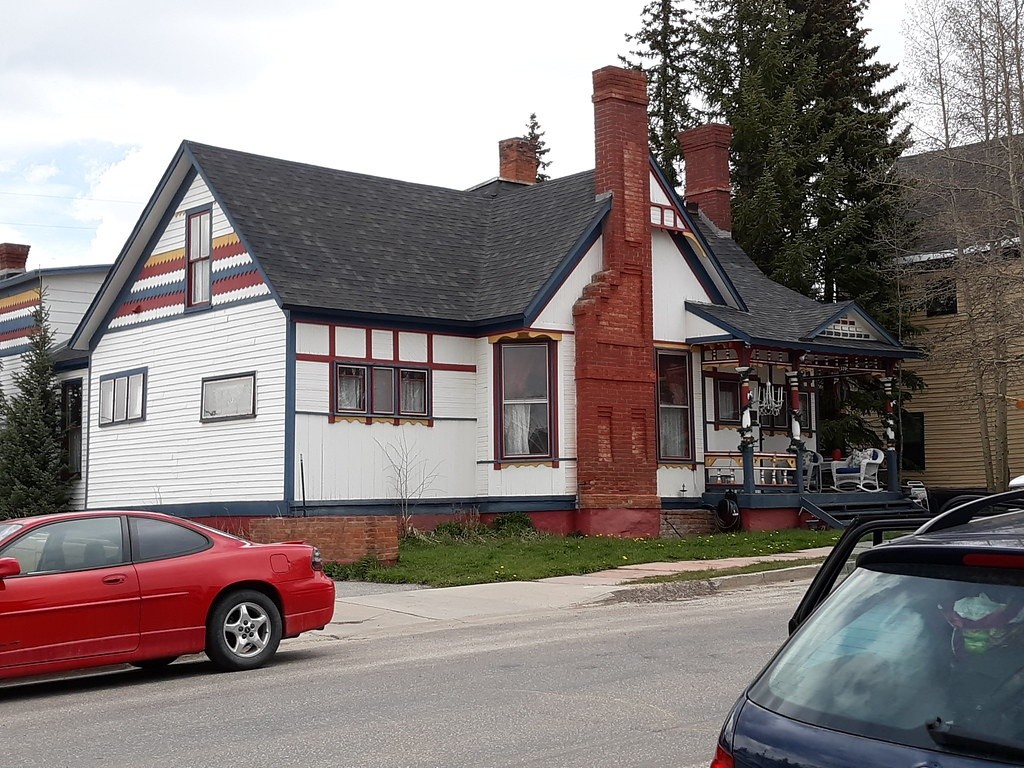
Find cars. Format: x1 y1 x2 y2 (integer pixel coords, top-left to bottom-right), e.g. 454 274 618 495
709 488 1023 768
0 512 336 680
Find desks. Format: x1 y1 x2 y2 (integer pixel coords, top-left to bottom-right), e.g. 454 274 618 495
818 457 861 492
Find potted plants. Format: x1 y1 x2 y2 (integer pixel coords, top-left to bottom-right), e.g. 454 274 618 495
839 402 849 414
818 417 852 461
884 393 896 413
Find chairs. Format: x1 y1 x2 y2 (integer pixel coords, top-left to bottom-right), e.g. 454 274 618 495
39 547 64 571
907 481 930 514
832 447 883 492
80 542 109 567
